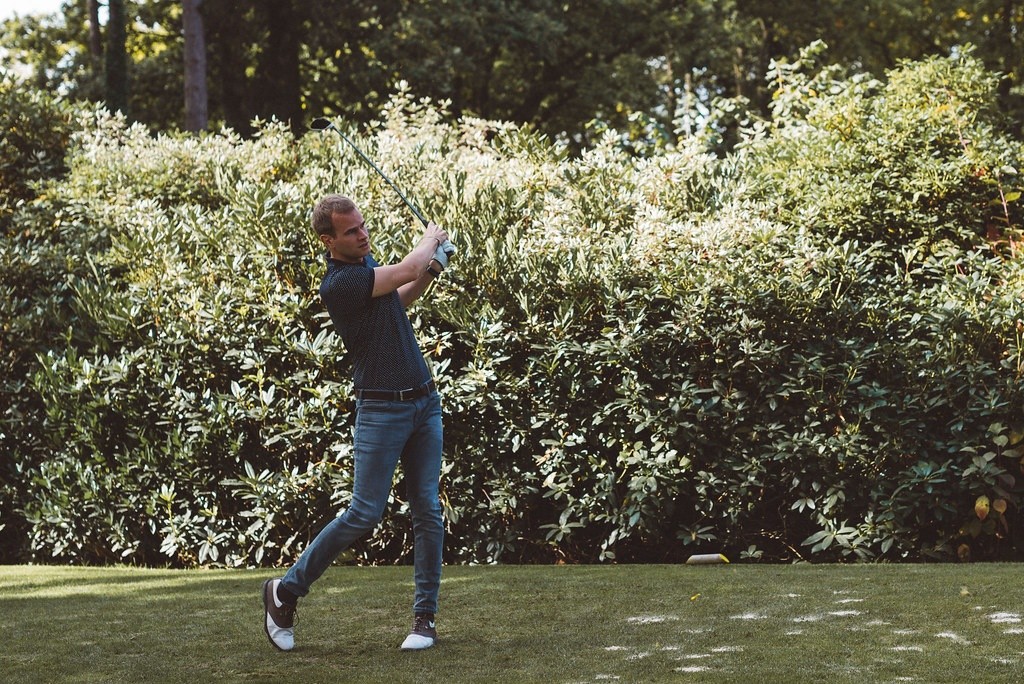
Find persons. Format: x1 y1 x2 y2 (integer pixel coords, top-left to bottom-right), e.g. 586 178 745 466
264 195 457 651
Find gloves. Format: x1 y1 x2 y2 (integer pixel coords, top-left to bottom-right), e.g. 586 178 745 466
431 239 455 271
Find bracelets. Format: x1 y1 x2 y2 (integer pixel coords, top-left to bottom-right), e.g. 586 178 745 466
426 265 439 278
432 238 440 248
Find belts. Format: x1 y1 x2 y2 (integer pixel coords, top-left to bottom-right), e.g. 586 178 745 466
354 377 436 402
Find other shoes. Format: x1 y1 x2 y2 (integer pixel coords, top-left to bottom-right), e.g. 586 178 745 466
400 613 438 651
263 578 299 651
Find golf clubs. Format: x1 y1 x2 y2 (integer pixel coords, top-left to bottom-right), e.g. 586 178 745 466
309 118 457 262
